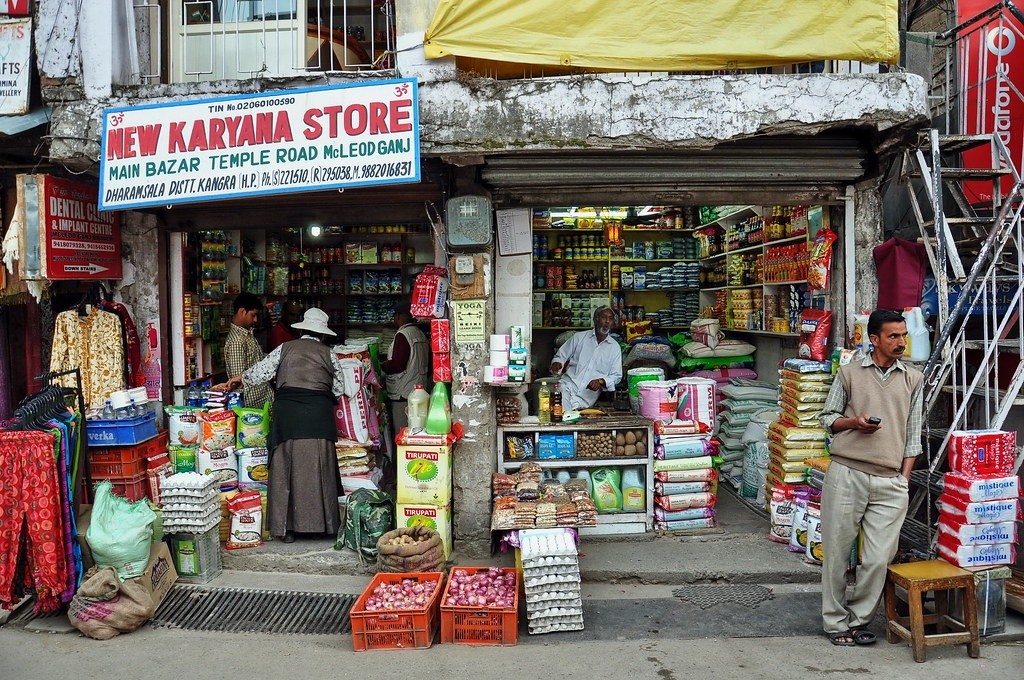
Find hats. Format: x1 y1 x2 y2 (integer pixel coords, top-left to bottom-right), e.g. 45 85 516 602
290 308 337 336
594 305 615 320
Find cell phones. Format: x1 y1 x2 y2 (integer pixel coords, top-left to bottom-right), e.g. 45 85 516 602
867 417 882 426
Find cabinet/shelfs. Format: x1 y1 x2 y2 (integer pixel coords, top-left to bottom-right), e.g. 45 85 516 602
171 225 435 408
494 205 836 534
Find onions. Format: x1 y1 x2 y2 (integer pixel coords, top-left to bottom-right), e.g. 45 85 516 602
444 566 515 641
364 580 437 643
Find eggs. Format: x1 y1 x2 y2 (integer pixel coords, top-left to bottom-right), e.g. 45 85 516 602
521 533 580 632
161 471 220 531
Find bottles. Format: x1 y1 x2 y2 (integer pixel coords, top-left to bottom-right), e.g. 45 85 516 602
853 307 931 361
699 204 811 288
408 385 431 430
103 399 147 419
289 247 344 321
185 381 211 407
533 232 645 328
538 380 551 423
378 240 416 264
550 383 563 422
665 206 697 230
622 466 646 512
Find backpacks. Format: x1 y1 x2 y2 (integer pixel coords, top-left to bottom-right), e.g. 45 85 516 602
334 488 394 566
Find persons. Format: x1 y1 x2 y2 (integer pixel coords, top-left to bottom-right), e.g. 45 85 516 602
551 305 622 412
227 307 345 543
820 310 924 647
381 303 429 446
223 297 273 411
271 303 306 351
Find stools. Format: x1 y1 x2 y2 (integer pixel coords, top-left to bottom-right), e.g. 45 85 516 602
885 559 980 663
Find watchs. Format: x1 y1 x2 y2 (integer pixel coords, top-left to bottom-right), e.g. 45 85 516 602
598 378 604 385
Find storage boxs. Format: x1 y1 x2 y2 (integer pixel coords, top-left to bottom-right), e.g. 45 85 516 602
440 567 520 647
76 412 223 613
396 443 452 559
349 572 444 653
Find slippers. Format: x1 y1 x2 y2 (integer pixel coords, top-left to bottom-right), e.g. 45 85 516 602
849 625 877 644
825 630 855 646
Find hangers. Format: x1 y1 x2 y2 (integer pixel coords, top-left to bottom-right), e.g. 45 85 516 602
69 283 103 316
0 371 70 432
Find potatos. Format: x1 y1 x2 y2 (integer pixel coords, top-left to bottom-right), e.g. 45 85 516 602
386 534 430 545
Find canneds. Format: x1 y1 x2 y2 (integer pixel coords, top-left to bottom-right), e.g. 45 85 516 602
532 233 609 260
406 246 415 263
764 285 791 333
610 291 644 327
289 246 343 295
351 224 421 233
